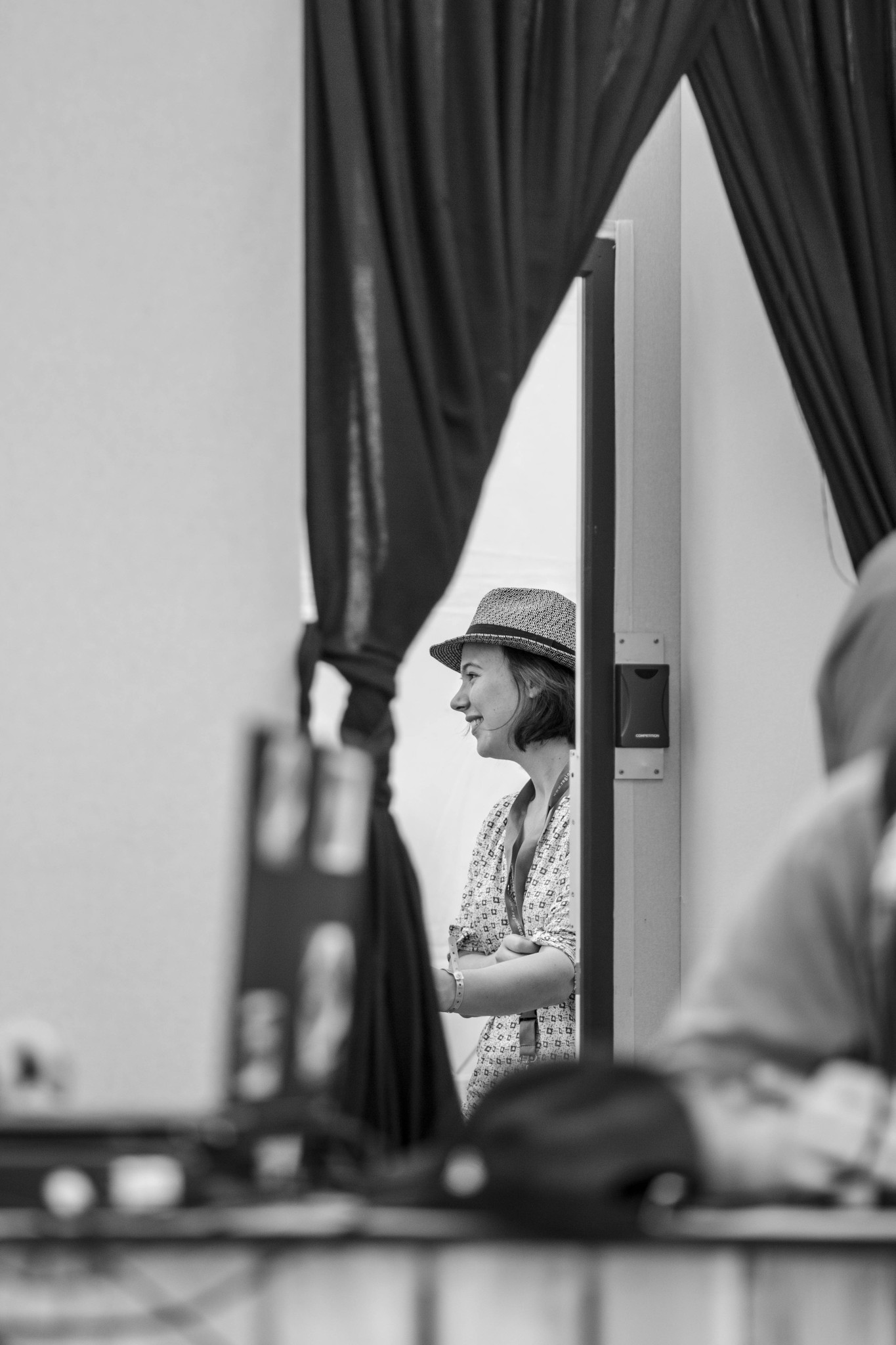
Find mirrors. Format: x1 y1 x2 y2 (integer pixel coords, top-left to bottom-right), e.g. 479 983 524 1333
383 223 614 1119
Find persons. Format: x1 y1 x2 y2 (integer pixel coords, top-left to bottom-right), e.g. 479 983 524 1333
638 532 896 1216
424 587 577 1119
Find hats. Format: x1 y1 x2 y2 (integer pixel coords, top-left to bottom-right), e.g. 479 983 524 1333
429 588 575 674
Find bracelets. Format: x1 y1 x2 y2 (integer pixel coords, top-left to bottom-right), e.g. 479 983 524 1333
441 936 465 1015
